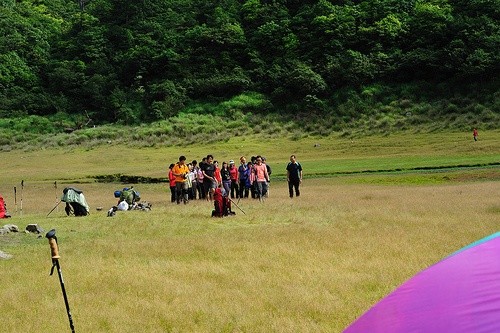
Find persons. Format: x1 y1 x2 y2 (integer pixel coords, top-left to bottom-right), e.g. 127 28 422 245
169 154 272 205
114 188 141 206
473 129 478 141
285 155 303 199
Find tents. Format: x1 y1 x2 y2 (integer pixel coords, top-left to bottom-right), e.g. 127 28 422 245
342 230 500 333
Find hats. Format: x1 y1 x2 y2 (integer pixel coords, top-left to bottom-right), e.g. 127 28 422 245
229 160 234 163
261 157 266 161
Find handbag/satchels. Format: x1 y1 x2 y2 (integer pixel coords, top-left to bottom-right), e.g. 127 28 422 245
185 173 193 188
